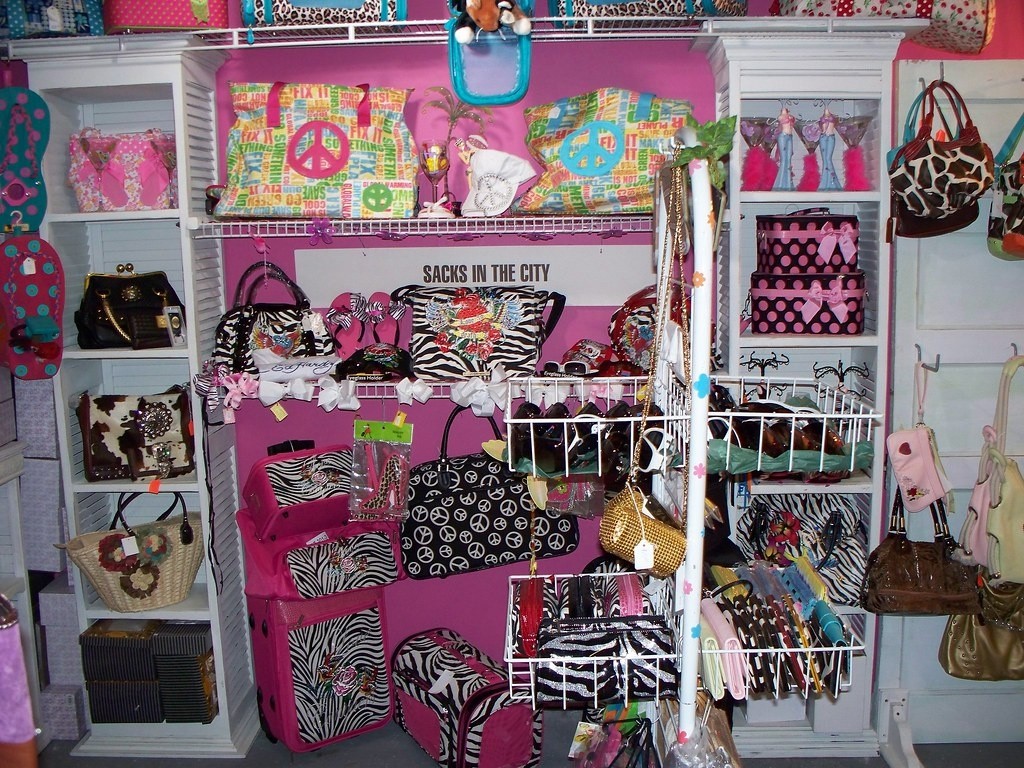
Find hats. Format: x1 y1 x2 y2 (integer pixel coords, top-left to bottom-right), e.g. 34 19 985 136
460 148 537 217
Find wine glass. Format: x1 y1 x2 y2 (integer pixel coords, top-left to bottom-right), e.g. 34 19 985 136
420 140 450 203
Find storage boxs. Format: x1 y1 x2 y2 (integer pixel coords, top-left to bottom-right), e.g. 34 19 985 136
62 508 74 586
86 681 166 723
79 619 167 681
41 685 87 740
0 367 13 403
39 576 79 627
19 457 67 572
45 626 86 686
0 398 16 446
151 620 219 725
15 377 59 459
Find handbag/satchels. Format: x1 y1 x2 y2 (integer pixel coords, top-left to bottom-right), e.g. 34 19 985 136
76 381 196 494
549 0 749 34
52 492 205 614
241 0 409 39
508 86 695 216
0 84 51 235
887 79 997 245
0 0 105 41
73 263 187 349
103 0 239 39
887 85 981 238
0 594 39 768
192 208 1022 768
0 235 63 381
769 0 997 57
987 105 1024 261
205 80 420 218
66 126 179 213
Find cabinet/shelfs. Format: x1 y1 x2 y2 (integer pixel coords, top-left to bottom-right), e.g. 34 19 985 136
0 0 929 768
